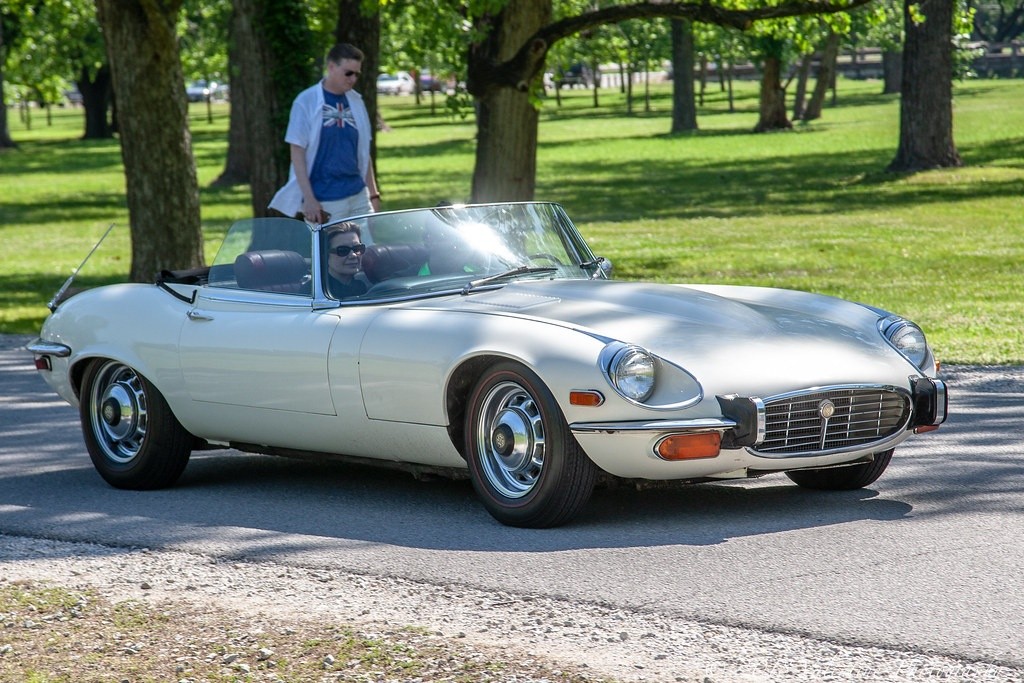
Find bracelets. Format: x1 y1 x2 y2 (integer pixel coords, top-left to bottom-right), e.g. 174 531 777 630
371 193 379 199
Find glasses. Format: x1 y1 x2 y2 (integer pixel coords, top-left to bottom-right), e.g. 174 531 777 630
337 64 361 78
327 244 365 257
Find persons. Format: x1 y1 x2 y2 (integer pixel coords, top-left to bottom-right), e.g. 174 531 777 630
300 221 367 298
416 198 473 276
266 44 380 246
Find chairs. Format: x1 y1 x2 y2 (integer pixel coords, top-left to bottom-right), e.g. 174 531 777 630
235 248 311 296
360 244 430 284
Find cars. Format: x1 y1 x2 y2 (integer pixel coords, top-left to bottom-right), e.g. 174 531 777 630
26 200 948 530
376 71 414 95
551 61 602 88
187 78 219 103
418 68 456 92
